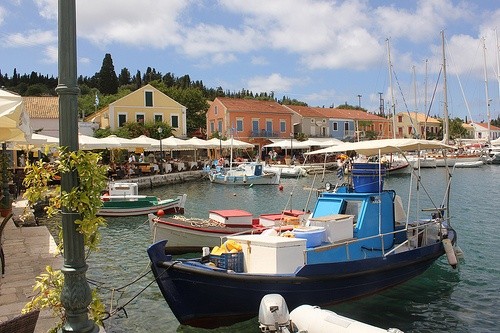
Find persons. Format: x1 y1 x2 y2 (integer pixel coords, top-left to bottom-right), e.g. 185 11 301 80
139 155 143 162
272 149 278 160
140 152 144 158
268 150 273 160
128 155 133 164
131 153 135 161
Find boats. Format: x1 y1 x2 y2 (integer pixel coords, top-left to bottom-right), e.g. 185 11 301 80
148 205 312 256
264 161 338 177
336 157 406 174
147 137 459 330
207 135 282 185
258 294 405 333
84 181 186 217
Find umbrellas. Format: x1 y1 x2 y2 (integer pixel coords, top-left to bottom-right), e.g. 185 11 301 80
47 133 256 161
0 130 47 209
0 89 32 146
263 140 348 156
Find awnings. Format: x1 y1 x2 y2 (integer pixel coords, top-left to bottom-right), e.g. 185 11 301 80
269 138 297 142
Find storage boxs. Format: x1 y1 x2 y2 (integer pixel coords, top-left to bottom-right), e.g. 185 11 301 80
308 214 356 243
293 226 327 248
227 236 307 274
208 252 243 273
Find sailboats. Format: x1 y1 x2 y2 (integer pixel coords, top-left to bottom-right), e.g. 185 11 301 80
377 28 500 168
